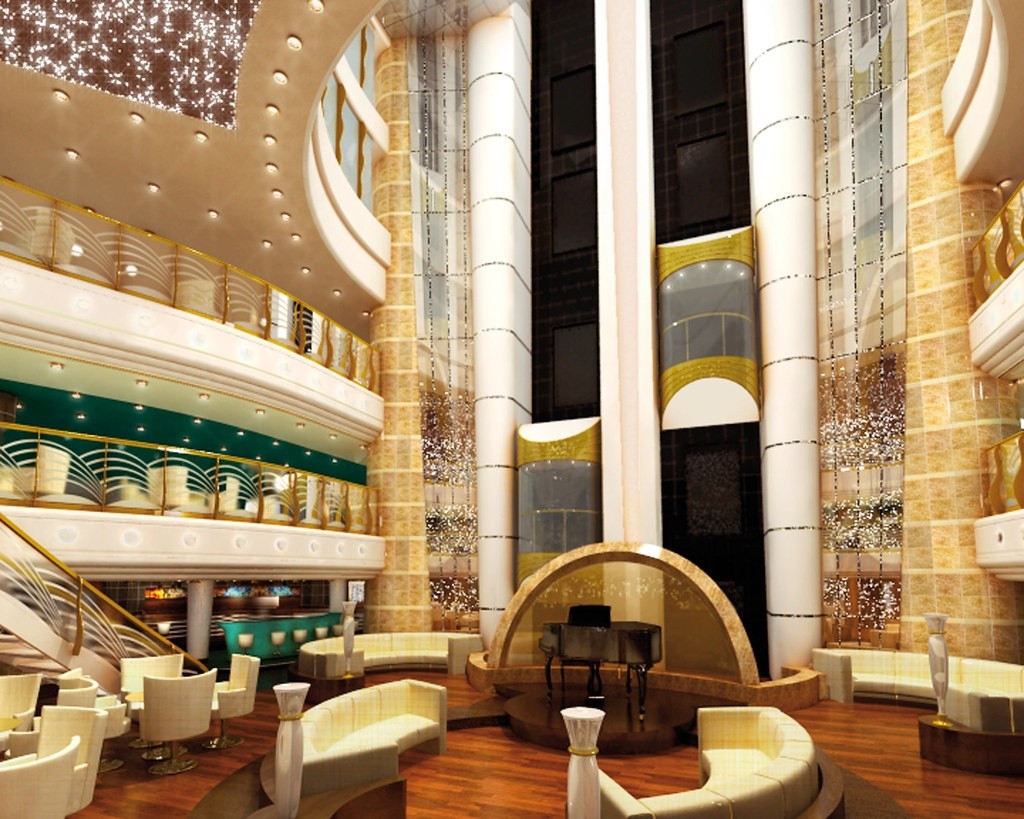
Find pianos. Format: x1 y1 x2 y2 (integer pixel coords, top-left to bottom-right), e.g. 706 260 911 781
539 604 663 722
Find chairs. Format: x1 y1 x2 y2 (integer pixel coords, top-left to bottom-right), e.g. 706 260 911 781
122 655 186 744
140 666 220 776
202 651 264 750
1 735 83 818
55 675 99 706
0 671 42 752
35 705 109 813
97 695 131 773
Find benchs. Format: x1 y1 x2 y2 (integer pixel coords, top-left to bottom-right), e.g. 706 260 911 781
810 646 1024 733
296 630 484 678
289 678 449 794
588 706 818 819
